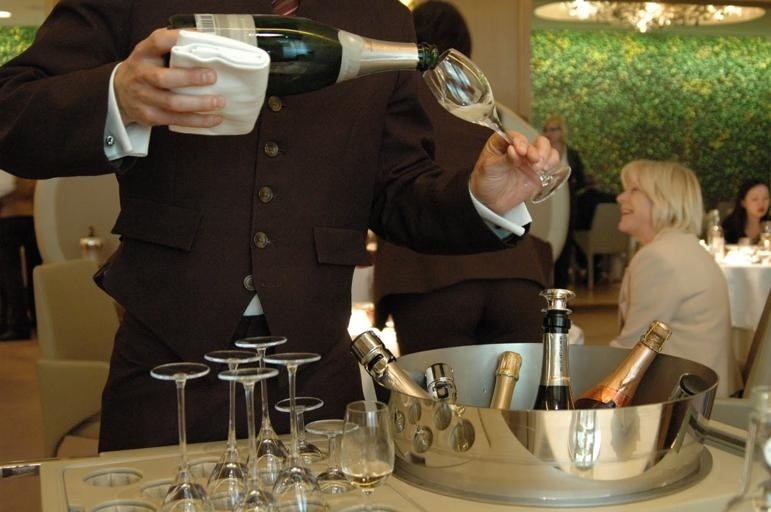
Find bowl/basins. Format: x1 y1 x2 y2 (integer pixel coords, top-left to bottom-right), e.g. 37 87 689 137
374 341 717 509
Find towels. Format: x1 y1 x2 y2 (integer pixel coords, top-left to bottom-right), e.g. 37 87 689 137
168 29 271 136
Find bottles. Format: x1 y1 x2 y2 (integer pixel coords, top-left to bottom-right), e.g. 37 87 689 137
760 216 771 251
705 208 726 264
422 361 459 405
531 289 580 411
576 319 673 412
668 373 708 398
723 382 771 512
169 13 440 102
351 328 432 400
488 350 526 411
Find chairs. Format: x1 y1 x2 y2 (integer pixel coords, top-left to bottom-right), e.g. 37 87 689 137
574 203 637 290
34 259 120 456
738 289 771 400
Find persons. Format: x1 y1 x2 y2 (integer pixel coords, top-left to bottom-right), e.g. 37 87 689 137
722 179 770 244
371 224 553 350
608 158 744 399
543 114 588 287
0 1 556 452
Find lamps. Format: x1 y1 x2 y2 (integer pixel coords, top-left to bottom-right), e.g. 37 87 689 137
535 0 765 33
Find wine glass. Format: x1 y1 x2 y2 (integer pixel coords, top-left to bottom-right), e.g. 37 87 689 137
421 47 573 205
149 334 395 511
